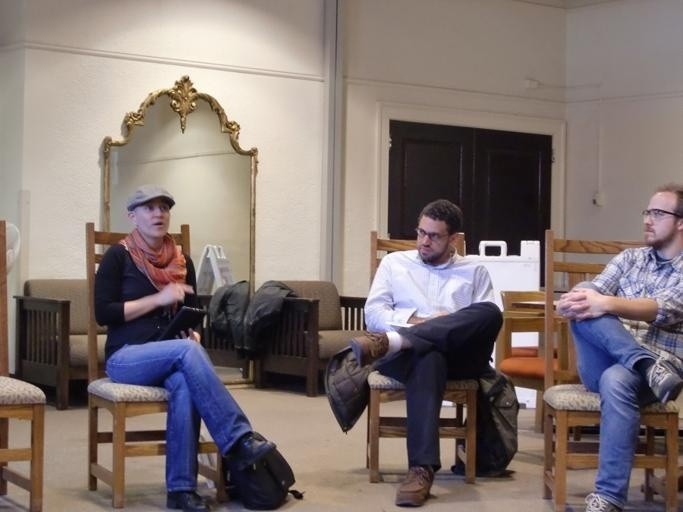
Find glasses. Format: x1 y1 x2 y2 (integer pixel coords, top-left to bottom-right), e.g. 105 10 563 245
414 228 452 240
641 208 683 218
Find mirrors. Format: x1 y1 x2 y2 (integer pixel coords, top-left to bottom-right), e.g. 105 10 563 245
98 70 258 389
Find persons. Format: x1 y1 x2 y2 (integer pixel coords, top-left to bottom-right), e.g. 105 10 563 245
554 178 682 511
350 199 505 507
92 187 274 511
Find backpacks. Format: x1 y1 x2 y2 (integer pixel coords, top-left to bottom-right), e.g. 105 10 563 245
224 430 296 509
463 372 521 477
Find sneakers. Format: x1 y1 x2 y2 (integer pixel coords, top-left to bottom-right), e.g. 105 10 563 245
349 332 391 368
583 492 627 511
645 358 682 405
395 466 435 507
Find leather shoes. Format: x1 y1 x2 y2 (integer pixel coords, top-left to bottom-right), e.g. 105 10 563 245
166 489 212 510
230 435 275 470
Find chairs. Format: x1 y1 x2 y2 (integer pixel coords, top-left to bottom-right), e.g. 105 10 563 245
85 223 233 510
189 277 250 379
0 219 51 512
11 274 109 410
252 278 376 399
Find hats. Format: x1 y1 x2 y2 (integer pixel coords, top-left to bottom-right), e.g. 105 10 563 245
125 184 175 210
